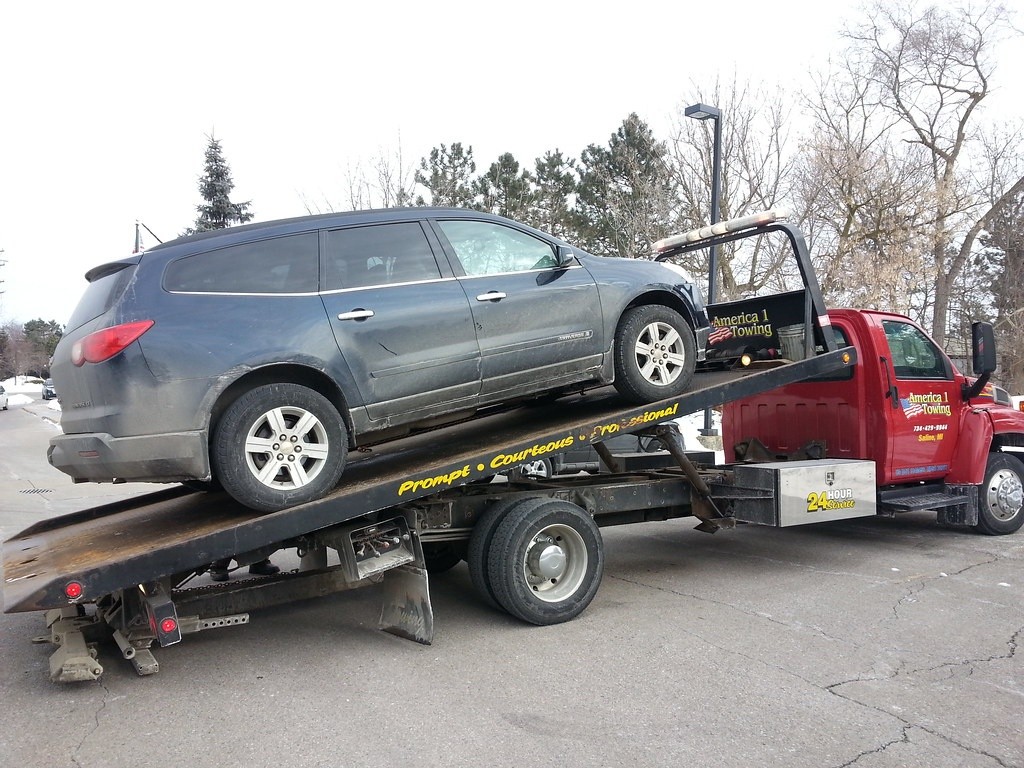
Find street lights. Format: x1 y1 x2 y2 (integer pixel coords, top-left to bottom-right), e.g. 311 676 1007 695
681 101 724 442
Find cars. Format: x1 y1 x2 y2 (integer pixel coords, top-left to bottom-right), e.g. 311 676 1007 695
0 376 58 411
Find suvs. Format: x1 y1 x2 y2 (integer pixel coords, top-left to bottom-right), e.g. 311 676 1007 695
46 203 711 513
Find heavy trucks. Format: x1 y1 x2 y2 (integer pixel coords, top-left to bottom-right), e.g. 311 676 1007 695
0 211 1024 687
